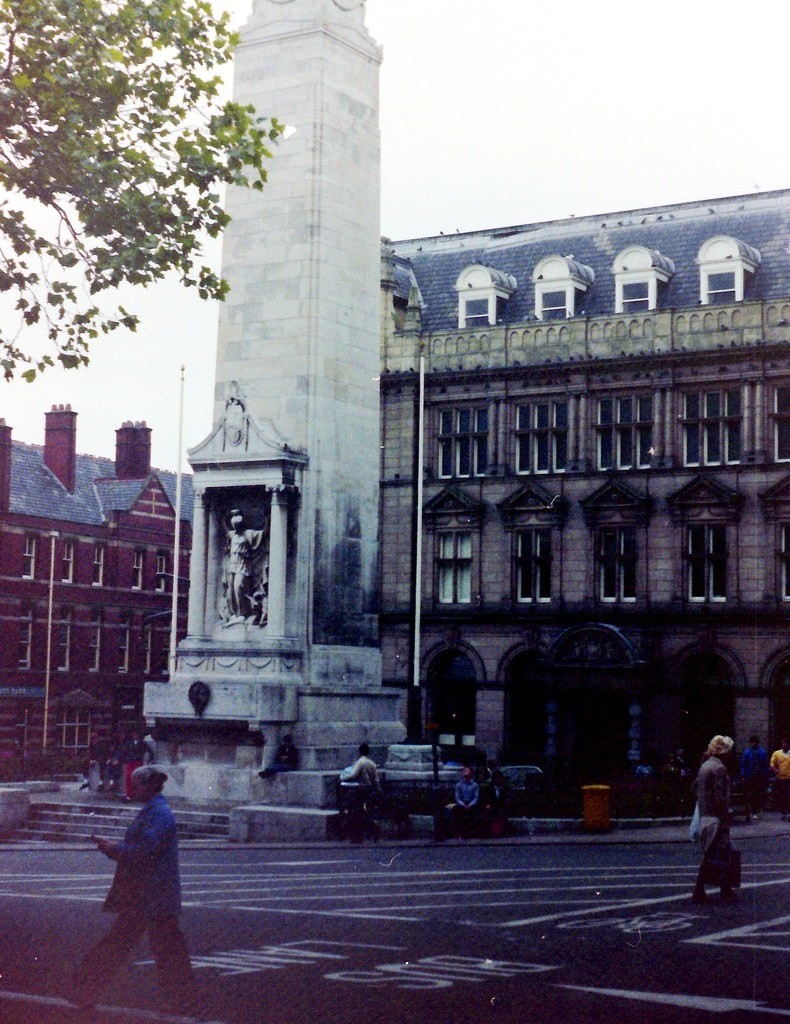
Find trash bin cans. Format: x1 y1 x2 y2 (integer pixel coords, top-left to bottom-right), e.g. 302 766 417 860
581 785 611 831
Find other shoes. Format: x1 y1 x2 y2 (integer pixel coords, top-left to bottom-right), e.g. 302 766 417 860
154 993 210 1014
373 831 384 843
752 810 763 820
353 835 364 843
79 781 90 790
259 768 272 779
123 796 135 804
692 892 716 904
781 815 790 823
96 783 117 791
60 982 96 1007
720 894 743 908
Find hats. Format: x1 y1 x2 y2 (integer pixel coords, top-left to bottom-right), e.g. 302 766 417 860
707 735 734 755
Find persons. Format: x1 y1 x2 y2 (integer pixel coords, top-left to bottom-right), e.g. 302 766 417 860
740 735 790 824
65 764 200 1016
78 729 154 803
455 766 510 837
221 509 269 622
692 735 734 905
635 749 709 788
258 735 299 779
338 743 384 845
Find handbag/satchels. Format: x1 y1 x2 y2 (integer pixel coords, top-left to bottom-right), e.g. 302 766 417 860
689 802 704 842
